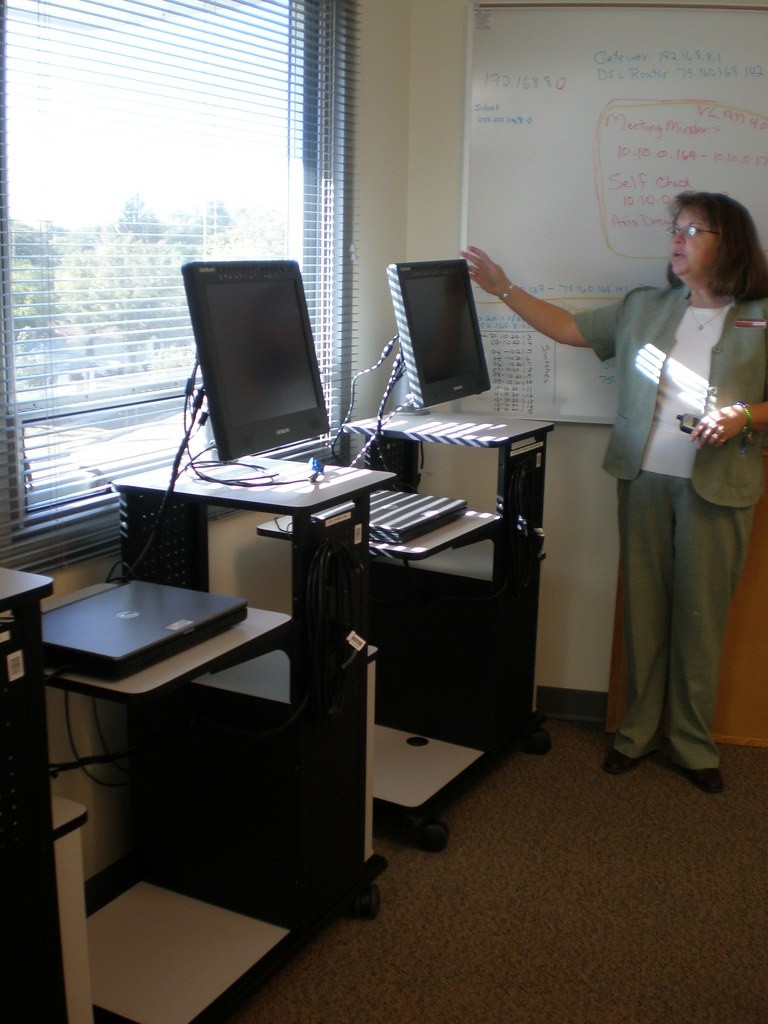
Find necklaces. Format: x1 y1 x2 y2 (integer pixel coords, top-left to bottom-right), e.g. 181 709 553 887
687 302 730 330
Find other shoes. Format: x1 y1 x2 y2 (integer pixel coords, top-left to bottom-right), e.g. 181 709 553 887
600 743 660 774
669 754 724 793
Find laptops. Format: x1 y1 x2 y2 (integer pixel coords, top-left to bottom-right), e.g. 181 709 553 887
310 489 468 546
42 580 248 679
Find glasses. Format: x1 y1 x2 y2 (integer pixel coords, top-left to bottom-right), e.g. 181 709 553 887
666 226 719 238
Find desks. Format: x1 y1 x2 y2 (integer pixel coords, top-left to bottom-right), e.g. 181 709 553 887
0 566 94 1024
256 411 555 854
43 453 388 1024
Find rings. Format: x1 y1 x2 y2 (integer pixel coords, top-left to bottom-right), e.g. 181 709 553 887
713 434 719 440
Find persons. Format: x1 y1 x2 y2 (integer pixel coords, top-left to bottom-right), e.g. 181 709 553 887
457 191 768 792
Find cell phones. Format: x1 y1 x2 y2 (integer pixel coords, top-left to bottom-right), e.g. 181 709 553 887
677 413 704 436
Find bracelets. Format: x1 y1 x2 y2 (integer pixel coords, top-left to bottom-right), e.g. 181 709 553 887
501 284 514 300
736 401 753 448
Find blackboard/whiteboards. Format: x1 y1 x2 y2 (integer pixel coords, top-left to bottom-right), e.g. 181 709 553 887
459 0 768 424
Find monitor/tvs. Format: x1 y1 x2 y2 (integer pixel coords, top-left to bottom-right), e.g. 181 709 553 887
386 259 491 410
181 261 330 462
